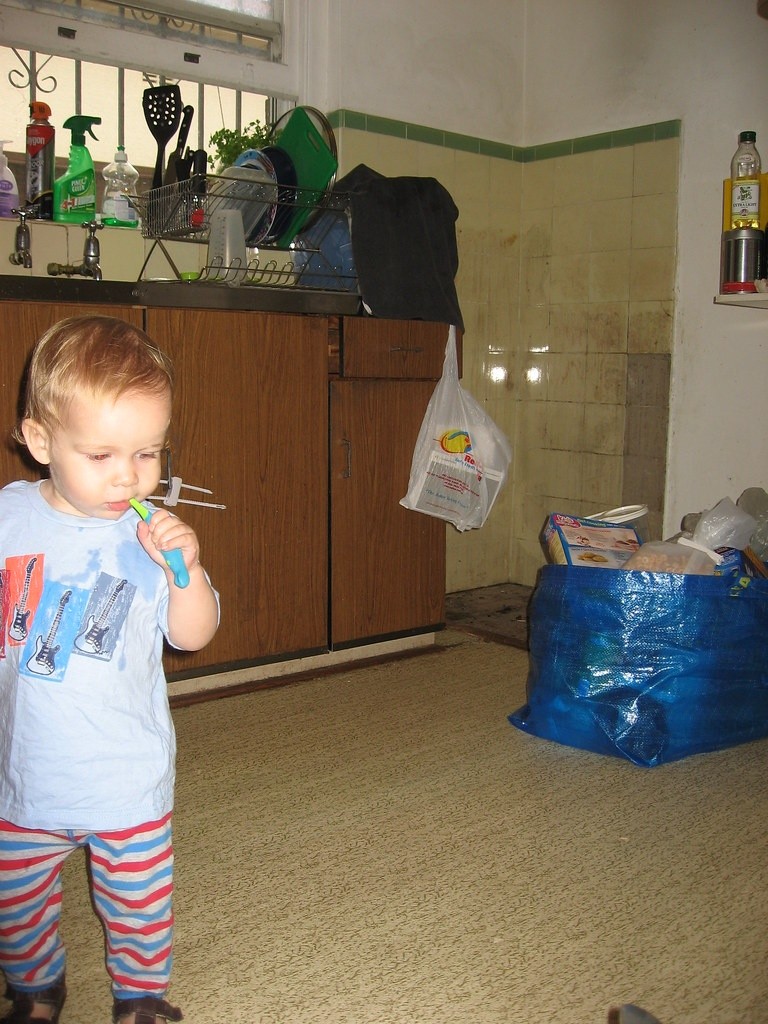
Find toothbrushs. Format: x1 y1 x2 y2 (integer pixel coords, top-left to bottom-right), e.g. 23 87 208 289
128 497 191 590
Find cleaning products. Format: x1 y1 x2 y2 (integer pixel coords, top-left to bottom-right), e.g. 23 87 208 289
100 145 140 229
52 115 102 226
0 139 21 219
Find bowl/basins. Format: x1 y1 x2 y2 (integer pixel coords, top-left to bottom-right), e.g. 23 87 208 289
180 271 200 280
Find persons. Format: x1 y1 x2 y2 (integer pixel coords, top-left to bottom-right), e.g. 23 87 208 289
0 314 223 1024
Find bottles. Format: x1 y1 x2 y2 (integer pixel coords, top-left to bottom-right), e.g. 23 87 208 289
730 131 761 228
101 144 139 228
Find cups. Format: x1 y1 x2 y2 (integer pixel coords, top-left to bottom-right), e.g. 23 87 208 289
206 209 249 279
724 227 764 294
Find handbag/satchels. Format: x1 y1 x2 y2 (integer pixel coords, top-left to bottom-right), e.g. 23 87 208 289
398 323 512 532
507 565 768 767
328 163 464 331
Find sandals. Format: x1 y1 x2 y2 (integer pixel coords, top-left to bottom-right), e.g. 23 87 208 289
113 993 184 1024
6 972 67 1024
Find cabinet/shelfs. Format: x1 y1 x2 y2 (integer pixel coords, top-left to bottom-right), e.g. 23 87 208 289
329 313 464 675
715 292 768 311
118 172 353 293
0 298 329 709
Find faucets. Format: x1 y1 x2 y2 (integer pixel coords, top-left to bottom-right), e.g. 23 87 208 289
8 208 34 269
77 219 105 280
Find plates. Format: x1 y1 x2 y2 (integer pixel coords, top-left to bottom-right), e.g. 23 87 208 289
234 149 278 245
261 146 297 243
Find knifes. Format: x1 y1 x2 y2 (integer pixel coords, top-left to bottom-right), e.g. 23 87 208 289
165 104 194 185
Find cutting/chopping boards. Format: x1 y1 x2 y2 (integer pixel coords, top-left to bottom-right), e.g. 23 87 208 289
276 106 338 248
